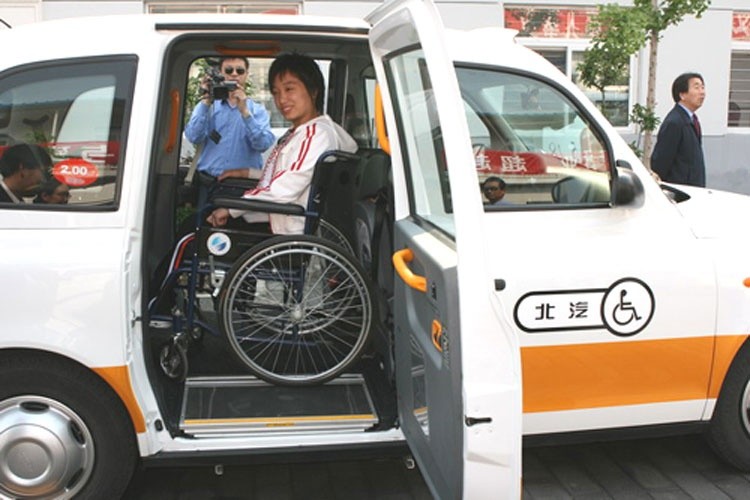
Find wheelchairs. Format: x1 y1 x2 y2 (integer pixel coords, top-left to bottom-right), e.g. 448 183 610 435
146 146 392 388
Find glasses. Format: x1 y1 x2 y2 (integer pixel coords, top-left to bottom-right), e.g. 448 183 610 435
224 65 246 75
37 167 54 178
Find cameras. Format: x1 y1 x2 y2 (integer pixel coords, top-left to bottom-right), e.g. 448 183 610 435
203 71 239 101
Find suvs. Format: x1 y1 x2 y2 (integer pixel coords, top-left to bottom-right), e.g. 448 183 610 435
0 0 750 500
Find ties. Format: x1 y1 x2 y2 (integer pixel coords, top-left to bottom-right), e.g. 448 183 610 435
692 113 701 139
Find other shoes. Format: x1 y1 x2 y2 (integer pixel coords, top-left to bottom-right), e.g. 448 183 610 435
148 301 182 328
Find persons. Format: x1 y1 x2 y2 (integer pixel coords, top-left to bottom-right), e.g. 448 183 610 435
728 91 740 126
141 54 359 329
650 73 708 190
0 143 72 205
481 176 517 204
